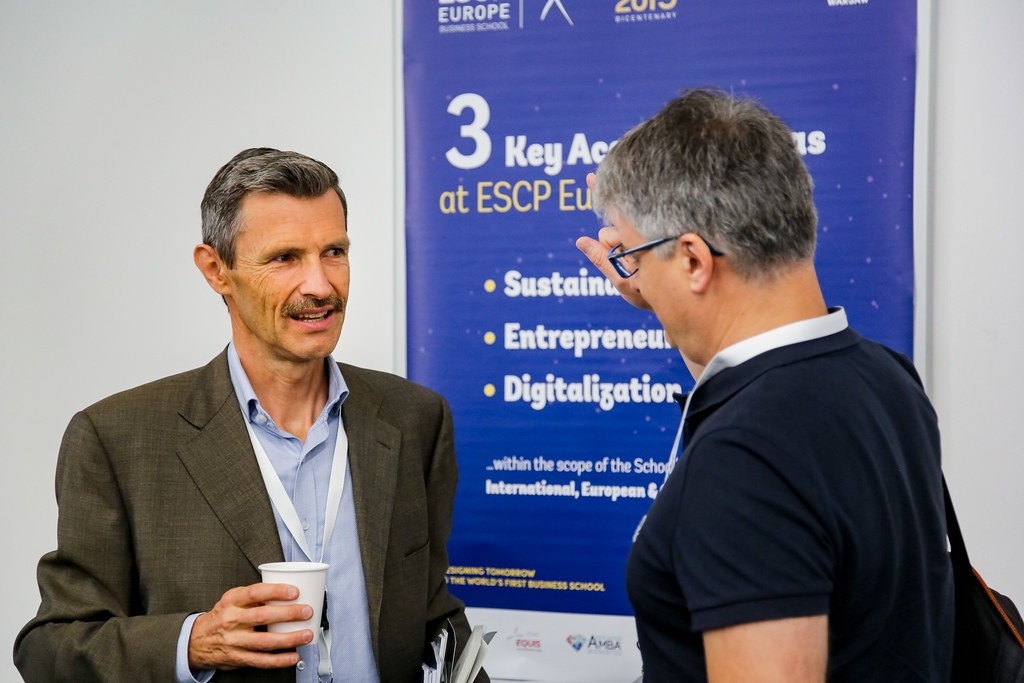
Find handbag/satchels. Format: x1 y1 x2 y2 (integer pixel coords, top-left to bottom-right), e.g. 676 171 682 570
949 550 1024 683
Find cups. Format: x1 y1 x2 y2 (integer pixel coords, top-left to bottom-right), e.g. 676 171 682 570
258 562 330 646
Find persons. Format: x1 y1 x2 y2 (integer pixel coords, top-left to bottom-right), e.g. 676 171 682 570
8 146 494 683
576 87 957 682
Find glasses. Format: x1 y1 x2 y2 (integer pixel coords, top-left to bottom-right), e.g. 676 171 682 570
609 235 723 280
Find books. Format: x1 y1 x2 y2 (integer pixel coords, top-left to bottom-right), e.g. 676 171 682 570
422 618 497 683
